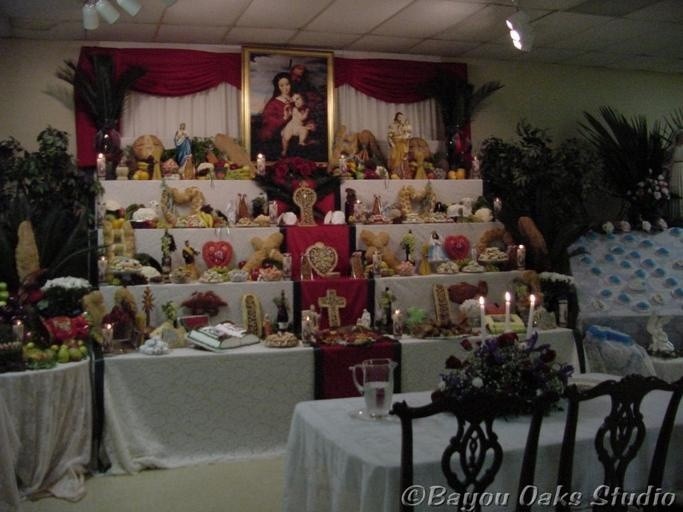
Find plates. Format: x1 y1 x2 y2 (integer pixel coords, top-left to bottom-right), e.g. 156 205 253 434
567 378 601 391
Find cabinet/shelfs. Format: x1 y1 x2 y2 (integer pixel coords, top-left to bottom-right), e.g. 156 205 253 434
96 180 581 475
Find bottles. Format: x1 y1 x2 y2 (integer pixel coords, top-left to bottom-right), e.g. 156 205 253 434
275 289 288 333
262 314 272 337
25 296 48 353
378 286 394 335
392 315 402 337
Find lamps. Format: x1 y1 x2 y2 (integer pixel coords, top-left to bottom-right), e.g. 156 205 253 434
502 0 537 56
80 0 142 32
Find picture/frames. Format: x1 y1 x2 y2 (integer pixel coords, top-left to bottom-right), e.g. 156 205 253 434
239 42 336 171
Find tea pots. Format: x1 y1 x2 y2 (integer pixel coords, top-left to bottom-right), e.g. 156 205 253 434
350 357 398 418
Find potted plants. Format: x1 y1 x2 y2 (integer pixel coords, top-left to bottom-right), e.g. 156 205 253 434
53 50 145 181
414 66 507 179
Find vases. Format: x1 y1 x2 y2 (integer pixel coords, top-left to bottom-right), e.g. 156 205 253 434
431 386 559 419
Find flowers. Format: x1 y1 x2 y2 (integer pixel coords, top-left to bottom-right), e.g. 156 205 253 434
438 331 574 421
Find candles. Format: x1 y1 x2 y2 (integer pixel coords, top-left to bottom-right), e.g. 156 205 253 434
516 244 526 270
301 315 311 348
391 307 402 340
12 320 23 342
493 197 501 221
97 257 107 286
501 291 511 334
101 320 113 357
478 296 487 346
525 294 536 337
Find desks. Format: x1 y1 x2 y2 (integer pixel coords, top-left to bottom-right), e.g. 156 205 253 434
279 371 683 512
0 355 95 511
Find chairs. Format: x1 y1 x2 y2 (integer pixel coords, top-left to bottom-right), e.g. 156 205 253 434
555 374 683 512
389 390 554 512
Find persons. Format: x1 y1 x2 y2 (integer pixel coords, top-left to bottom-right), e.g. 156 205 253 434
173 123 192 162
259 62 325 155
385 110 411 174
